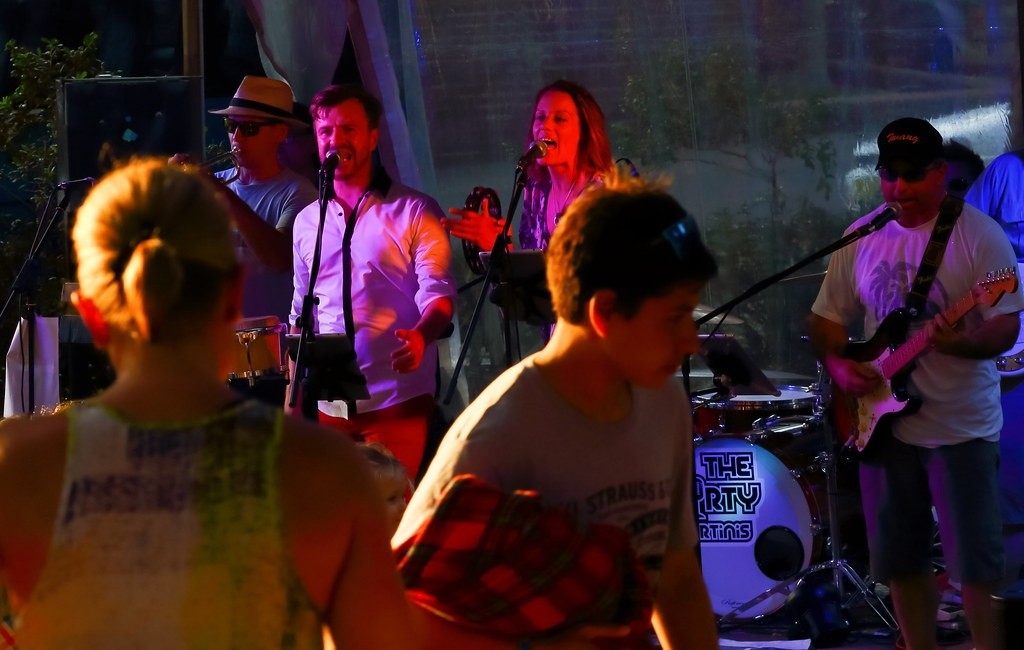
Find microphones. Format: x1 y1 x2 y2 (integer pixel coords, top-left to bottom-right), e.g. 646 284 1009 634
515 140 549 174
321 149 344 170
56 177 95 190
625 159 639 177
861 202 903 238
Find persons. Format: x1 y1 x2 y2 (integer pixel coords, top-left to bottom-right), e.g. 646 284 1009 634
162 73 320 408
440 81 615 349
0 157 512 650
384 165 721 650
278 80 457 488
354 438 418 538
817 115 1024 650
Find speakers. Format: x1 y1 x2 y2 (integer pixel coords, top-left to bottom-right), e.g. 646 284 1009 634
58 75 201 264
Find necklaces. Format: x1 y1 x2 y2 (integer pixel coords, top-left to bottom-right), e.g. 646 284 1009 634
550 179 575 226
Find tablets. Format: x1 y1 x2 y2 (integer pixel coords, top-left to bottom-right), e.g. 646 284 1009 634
479 250 559 322
697 334 780 396
286 333 371 400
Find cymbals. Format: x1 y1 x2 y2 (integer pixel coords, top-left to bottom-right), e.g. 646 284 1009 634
779 269 828 285
459 186 504 274
691 303 745 326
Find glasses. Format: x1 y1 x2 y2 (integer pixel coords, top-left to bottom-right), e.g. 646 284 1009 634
877 163 939 184
222 116 273 137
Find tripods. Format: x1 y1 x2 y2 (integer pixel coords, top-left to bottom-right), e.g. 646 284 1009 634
716 361 904 645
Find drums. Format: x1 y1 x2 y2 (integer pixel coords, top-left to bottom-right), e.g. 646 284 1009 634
223 315 291 383
693 384 832 440
693 434 826 631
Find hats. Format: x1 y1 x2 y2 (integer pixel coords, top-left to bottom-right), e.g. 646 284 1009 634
208 75 309 129
875 117 942 170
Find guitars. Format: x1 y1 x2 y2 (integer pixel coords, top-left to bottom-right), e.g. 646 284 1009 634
826 264 1020 464
